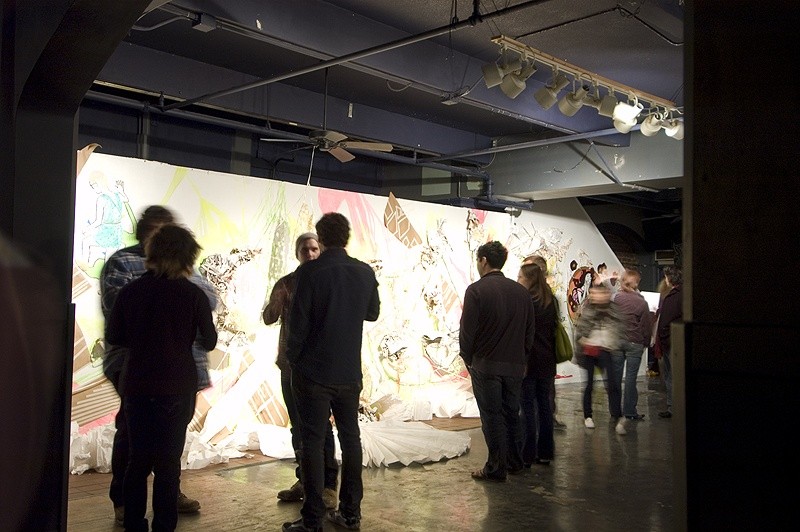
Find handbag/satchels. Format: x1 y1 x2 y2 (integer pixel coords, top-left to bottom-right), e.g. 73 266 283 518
551 297 573 363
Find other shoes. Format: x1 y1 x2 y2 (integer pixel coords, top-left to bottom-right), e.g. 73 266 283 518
626 413 644 420
471 467 506 482
584 417 596 430
609 416 618 428
506 468 523 474
658 411 672 417
552 418 567 430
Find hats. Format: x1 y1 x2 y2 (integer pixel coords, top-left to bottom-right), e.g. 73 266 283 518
295 234 321 258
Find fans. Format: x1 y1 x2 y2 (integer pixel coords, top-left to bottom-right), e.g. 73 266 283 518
259 67 392 165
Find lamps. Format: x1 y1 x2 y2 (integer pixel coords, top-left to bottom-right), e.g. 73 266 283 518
534 64 570 111
640 100 665 136
612 92 644 133
662 106 685 140
499 50 537 100
583 81 617 117
481 44 524 88
558 73 590 117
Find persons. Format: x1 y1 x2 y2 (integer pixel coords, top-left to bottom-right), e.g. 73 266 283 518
100 206 217 526
612 270 653 420
648 242 683 418
458 240 535 483
104 222 219 532
522 255 567 439
572 283 628 436
263 233 339 509
282 213 381 532
517 263 557 469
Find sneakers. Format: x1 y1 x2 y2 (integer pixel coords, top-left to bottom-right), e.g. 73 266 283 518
321 488 338 509
174 492 201 512
114 507 149 526
281 518 324 532
326 509 360 530
277 481 305 499
